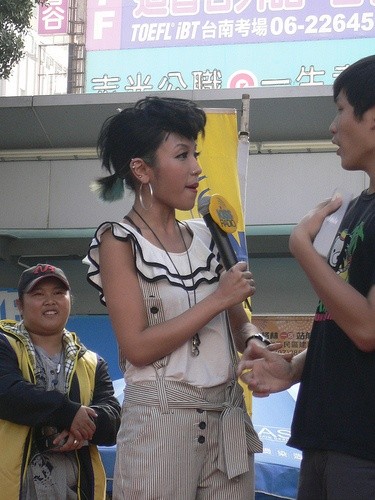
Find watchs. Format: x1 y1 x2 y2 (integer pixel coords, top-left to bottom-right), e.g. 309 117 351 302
246 333 272 347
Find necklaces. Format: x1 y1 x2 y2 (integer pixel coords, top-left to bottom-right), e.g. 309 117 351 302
132 204 201 358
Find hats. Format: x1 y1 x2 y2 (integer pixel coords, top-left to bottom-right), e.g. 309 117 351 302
18 263 71 300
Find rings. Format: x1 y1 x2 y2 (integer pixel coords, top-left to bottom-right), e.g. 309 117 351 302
73 438 80 447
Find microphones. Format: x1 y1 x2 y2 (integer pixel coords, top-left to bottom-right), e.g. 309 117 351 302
198 194 239 272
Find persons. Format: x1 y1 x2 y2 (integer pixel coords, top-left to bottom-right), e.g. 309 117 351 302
82 94 294 500
0 263 122 500
235 55 375 500
